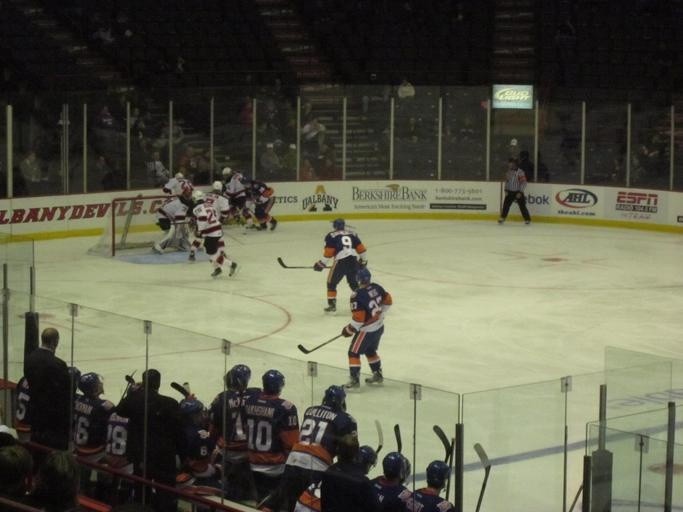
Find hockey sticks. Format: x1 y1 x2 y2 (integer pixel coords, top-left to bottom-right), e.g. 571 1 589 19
374 419 491 512
170 381 190 398
126 374 135 395
298 334 343 354
277 257 333 269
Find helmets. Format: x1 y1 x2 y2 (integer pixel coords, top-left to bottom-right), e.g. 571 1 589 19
427 461 448 492
176 398 209 425
333 218 345 230
221 167 233 178
356 268 371 284
213 180 223 192
192 191 206 203
323 384 346 409
67 367 104 397
358 445 377 471
224 364 251 392
262 369 284 395
383 452 412 486
175 172 183 179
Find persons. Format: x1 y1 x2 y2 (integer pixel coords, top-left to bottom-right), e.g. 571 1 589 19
342 266 393 388
0 327 458 511
1 55 683 275
315 217 366 311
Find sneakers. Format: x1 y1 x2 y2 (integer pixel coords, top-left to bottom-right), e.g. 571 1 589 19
211 267 221 276
229 262 236 275
155 244 164 253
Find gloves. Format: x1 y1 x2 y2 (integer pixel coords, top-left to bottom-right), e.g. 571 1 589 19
158 218 170 230
342 325 354 337
313 261 325 271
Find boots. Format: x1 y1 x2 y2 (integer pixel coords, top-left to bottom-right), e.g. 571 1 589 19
269 217 277 230
365 368 383 384
345 372 361 388
256 222 267 230
324 299 337 312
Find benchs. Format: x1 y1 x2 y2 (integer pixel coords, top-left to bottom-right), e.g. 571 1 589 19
0 0 682 200
